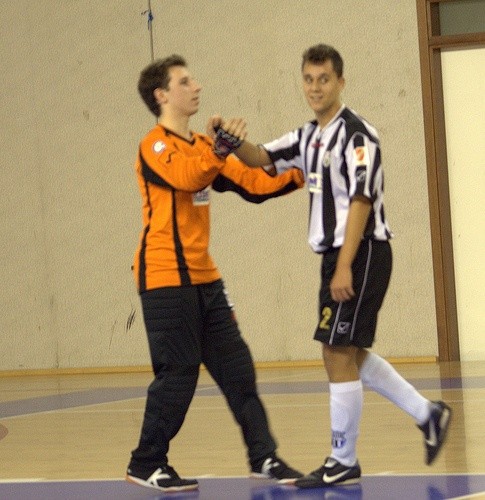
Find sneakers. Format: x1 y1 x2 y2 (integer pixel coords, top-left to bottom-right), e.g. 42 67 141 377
127 464 198 491
417 401 451 464
295 457 360 488
250 454 304 484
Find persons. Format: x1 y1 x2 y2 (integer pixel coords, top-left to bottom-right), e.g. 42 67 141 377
207 43 454 489
126 54 306 492
